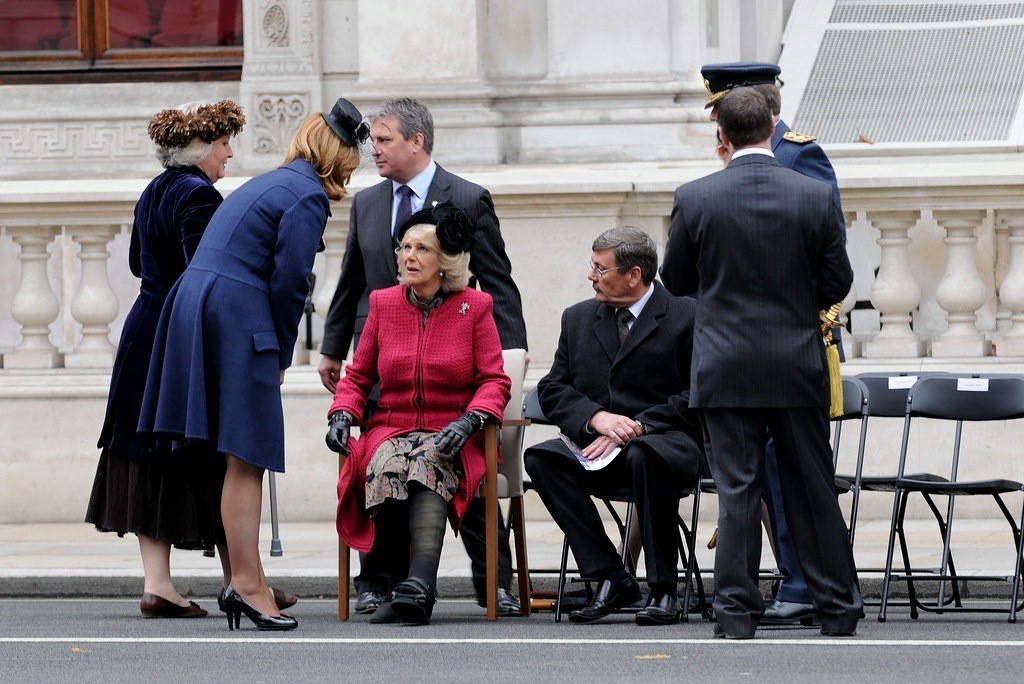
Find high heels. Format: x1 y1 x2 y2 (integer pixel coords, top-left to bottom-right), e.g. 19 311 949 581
222 587 297 631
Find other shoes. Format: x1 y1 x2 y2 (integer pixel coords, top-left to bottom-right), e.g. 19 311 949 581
713 623 754 639
368 599 392 623
391 577 433 624
551 583 597 617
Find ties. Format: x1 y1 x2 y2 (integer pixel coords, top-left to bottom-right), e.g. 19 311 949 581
392 185 414 255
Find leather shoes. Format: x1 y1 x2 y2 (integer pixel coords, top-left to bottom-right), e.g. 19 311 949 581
217 587 297 610
635 590 682 624
140 592 208 617
569 573 642 622
355 589 387 613
495 587 520 613
760 600 821 626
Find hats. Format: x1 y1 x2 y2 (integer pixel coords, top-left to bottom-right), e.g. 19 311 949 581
700 63 782 109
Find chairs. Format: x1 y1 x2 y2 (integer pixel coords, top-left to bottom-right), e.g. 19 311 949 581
333 346 1024 625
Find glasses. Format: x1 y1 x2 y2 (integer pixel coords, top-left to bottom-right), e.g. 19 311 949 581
589 261 634 276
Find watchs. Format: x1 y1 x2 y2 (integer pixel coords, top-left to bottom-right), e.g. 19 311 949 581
634 419 646 436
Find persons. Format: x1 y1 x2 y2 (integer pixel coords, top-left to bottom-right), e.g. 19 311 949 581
325 198 513 628
316 95 533 617
83 98 297 620
523 223 710 627
659 61 867 641
136 96 373 633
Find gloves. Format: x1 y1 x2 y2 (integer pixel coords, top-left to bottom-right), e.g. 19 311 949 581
434 411 480 455
327 413 351 456
